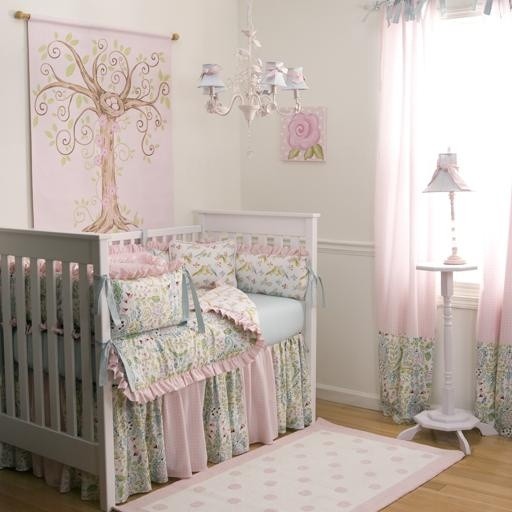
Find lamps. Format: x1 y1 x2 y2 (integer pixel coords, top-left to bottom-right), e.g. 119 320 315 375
195 0 310 159
423 152 472 264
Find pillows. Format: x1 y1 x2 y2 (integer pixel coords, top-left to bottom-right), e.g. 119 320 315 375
168 237 238 289
235 243 311 300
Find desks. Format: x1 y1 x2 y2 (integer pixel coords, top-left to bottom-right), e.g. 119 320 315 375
395 263 499 455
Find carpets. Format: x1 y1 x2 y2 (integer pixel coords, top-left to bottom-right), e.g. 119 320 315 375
111 417 465 512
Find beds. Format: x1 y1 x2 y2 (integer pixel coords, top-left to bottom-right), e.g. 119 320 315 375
1 206 321 511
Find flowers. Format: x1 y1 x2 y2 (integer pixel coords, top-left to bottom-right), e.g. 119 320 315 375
287 111 324 161
